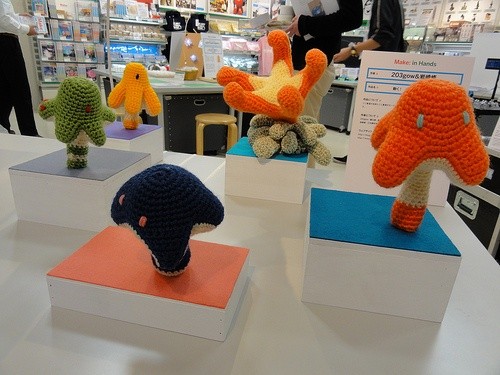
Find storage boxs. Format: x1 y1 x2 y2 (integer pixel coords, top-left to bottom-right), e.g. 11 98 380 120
446 137 500 261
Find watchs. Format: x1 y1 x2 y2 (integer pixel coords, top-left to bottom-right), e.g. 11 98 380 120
350 45 358 59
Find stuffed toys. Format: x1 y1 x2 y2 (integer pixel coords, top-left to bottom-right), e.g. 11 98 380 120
233 0 244 15
39 76 115 169
111 165 224 277
106 63 160 130
216 29 332 166
371 78 490 232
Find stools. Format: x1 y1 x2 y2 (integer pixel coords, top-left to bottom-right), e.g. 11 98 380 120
195 113 238 156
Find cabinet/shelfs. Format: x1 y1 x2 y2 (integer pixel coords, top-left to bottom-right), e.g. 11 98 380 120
319 79 357 134
24 0 271 93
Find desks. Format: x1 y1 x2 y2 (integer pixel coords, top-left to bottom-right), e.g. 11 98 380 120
89 68 255 159
0 133 500 375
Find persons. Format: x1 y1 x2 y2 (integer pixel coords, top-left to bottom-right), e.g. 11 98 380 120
0 0 45 138
284 0 363 71
332 0 405 68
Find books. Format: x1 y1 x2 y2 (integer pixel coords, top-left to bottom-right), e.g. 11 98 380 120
32 0 105 84
18 17 47 35
